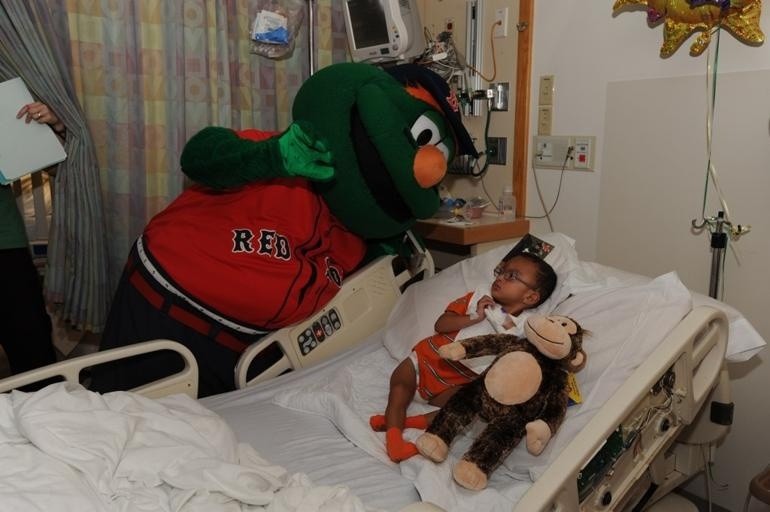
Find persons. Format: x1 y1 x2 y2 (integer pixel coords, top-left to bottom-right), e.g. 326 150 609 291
0 102 67 393
370 252 557 463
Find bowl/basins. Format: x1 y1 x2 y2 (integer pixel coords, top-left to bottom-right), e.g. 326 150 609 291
465 202 495 219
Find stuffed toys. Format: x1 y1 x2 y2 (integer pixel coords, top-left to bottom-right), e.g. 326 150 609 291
85 61 480 399
416 314 587 492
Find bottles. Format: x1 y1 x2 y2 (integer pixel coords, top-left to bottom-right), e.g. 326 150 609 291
499 186 517 221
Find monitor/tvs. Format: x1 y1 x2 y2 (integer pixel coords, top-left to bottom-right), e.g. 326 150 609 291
342 0 428 64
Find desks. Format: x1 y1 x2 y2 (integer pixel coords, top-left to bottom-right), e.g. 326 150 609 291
412 218 529 272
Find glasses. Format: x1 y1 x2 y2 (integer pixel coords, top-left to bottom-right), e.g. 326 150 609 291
493 267 534 291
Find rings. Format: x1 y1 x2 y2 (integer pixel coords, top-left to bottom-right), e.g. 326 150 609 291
38 112 40 118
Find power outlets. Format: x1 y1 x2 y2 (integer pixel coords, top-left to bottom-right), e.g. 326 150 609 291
494 8 507 37
532 136 595 173
489 83 508 111
485 137 507 165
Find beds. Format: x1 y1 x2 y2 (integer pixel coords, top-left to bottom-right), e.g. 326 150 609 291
0 235 765 512
